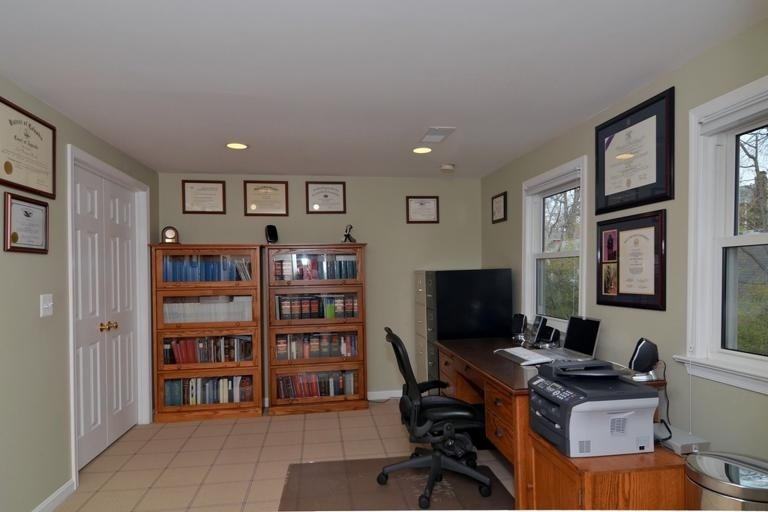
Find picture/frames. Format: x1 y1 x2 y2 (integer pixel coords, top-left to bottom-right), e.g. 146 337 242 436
491 191 507 223
182 180 226 215
306 180 347 215
5 191 49 255
592 84 675 218
0 96 58 202
406 195 440 225
594 208 669 314
242 179 290 217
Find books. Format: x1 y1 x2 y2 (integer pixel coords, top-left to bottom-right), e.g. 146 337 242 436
162 295 254 324
163 336 253 364
271 254 358 280
275 371 359 400
275 333 358 358
163 255 251 280
274 294 358 319
162 375 253 405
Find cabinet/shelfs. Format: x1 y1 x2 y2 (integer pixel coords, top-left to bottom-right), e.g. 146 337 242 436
149 242 263 424
263 242 371 417
527 429 690 511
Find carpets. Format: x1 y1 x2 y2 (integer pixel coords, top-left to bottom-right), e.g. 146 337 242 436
277 457 516 511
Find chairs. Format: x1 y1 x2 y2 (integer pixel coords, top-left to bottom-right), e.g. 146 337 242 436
377 325 492 508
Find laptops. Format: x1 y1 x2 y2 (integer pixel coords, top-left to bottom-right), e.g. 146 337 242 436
531 315 602 363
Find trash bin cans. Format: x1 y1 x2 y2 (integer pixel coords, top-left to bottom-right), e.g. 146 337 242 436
684 451 768 510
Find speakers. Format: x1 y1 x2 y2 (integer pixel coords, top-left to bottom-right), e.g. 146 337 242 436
528 316 548 346
265 224 278 241
511 313 527 333
541 325 561 344
628 337 659 373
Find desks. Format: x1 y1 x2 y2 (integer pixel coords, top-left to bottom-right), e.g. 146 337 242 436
438 342 666 511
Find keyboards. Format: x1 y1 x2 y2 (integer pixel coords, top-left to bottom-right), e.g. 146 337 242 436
493 346 553 367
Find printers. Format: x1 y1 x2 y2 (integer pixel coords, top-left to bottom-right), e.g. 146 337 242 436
528 359 659 458
